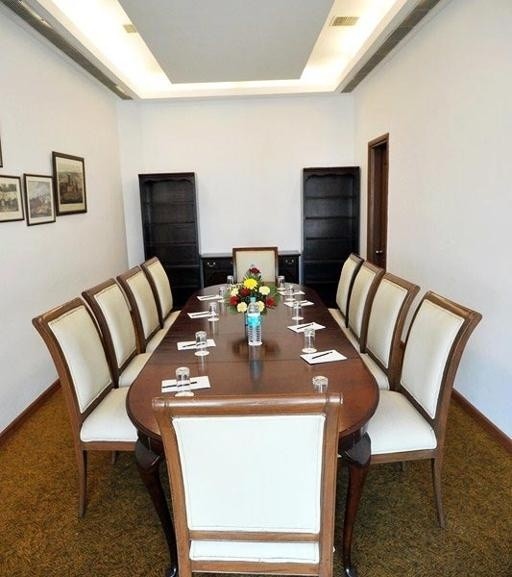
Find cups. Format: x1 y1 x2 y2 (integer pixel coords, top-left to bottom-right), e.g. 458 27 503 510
197 358 208 375
207 301 220 322
226 274 234 290
209 322 220 338
276 274 286 291
312 374 329 394
291 306 304 321
218 285 226 302
301 326 318 353
194 329 210 357
175 365 192 394
285 285 297 301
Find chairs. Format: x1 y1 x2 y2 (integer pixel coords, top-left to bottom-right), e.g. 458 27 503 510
116 266 169 352
327 252 365 328
81 277 153 389
140 256 183 329
149 390 343 577
341 290 484 566
31 296 139 518
232 246 279 283
338 261 386 353
357 272 422 474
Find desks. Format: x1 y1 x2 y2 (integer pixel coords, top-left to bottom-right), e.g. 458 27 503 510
125 282 380 577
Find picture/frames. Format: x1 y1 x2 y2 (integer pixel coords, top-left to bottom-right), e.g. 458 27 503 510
0 174 25 224
0 138 4 168
52 151 88 217
23 173 56 226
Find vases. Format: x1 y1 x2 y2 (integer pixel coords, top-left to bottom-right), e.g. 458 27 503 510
243 311 264 343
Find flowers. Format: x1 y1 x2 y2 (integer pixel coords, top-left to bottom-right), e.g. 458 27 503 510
222 264 283 317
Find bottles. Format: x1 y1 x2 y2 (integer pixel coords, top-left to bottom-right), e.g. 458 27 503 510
248 346 265 387
246 296 263 346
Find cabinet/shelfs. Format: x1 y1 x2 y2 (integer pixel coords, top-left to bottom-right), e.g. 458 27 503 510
199 250 301 289
137 171 202 310
301 165 362 309
